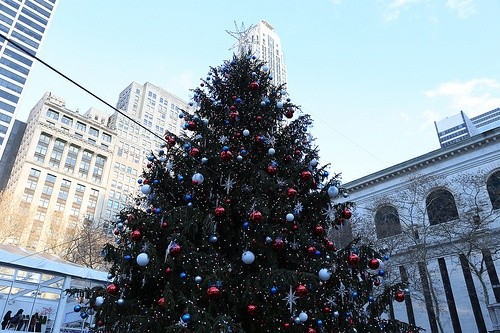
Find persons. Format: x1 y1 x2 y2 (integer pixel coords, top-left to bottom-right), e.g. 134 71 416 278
1 309 39 331
